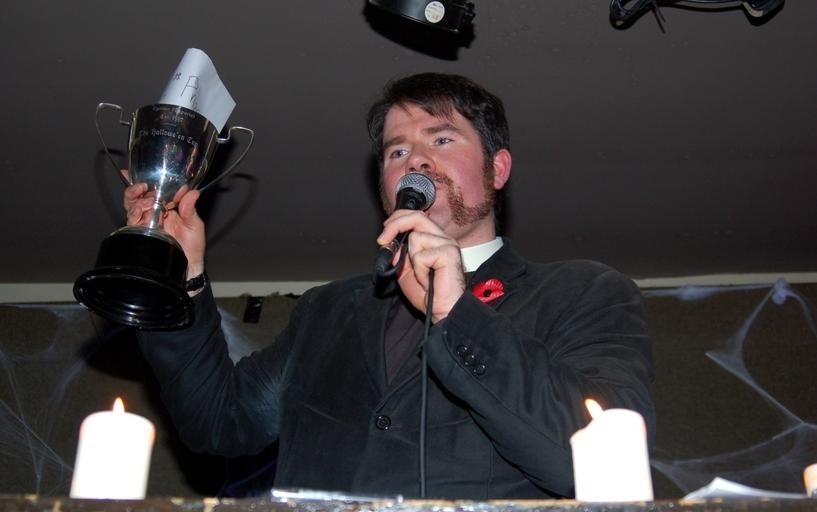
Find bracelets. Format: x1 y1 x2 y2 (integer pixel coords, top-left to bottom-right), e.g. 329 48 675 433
185 271 209 293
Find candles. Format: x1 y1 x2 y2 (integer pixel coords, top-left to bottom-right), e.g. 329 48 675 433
568 408 654 502
69 410 158 500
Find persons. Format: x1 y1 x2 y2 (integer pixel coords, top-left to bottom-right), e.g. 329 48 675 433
118 73 657 500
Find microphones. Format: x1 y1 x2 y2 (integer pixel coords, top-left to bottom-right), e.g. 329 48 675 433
373 173 437 272
